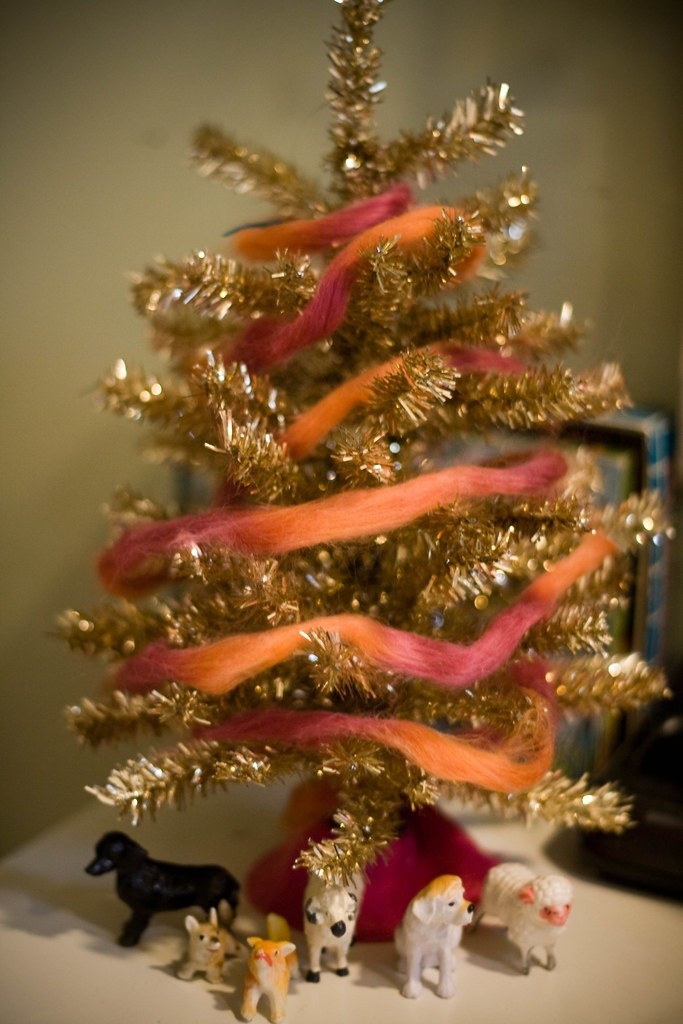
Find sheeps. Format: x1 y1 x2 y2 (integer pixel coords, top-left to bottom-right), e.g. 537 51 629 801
473 860 572 974
302 863 363 984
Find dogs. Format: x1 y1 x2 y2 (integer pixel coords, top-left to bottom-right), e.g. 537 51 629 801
239 911 298 1024
178 907 247 984
392 874 474 1001
84 829 241 948
